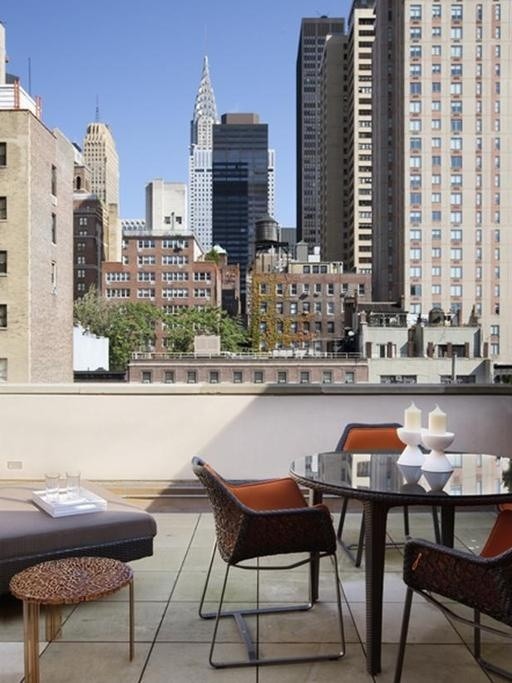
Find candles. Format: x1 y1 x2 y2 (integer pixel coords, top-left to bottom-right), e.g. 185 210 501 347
405 401 421 430
428 403 447 434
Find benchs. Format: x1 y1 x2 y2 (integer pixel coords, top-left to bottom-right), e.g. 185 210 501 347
0 482 156 626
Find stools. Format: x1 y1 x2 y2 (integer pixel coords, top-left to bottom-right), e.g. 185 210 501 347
11 558 134 683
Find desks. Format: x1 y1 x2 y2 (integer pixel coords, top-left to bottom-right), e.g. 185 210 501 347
287 449 510 675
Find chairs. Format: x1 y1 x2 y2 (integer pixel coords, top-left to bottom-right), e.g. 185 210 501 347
191 453 351 670
334 423 441 569
390 507 511 682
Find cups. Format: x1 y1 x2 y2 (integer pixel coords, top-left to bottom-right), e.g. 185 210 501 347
45 469 81 502
